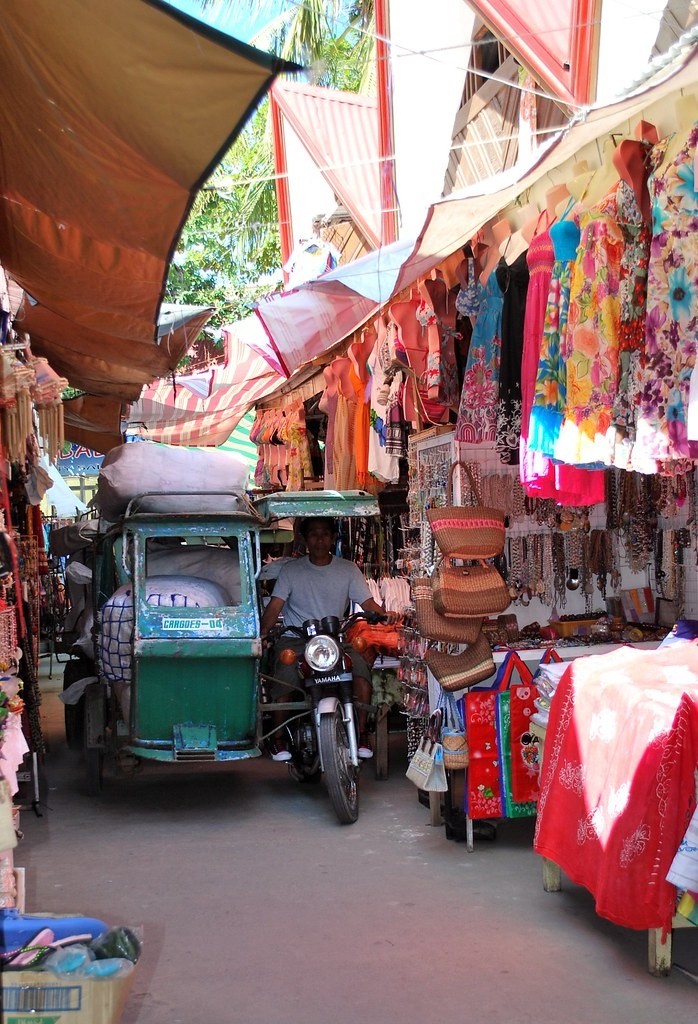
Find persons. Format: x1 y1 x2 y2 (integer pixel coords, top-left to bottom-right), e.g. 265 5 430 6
258 518 403 762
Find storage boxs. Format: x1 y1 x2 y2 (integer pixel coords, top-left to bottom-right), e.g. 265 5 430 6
550 620 597 638
0 912 144 1024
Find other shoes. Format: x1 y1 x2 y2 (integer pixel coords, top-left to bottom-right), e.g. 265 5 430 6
0 922 143 980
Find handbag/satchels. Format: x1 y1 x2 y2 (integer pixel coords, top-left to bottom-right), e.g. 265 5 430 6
406 460 510 792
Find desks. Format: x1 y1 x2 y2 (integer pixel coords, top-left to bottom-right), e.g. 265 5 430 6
369 655 402 781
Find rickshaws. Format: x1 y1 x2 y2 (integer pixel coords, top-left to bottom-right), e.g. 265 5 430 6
63 488 388 825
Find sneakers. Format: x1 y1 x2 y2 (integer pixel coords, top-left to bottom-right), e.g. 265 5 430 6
267 740 293 760
358 743 374 758
